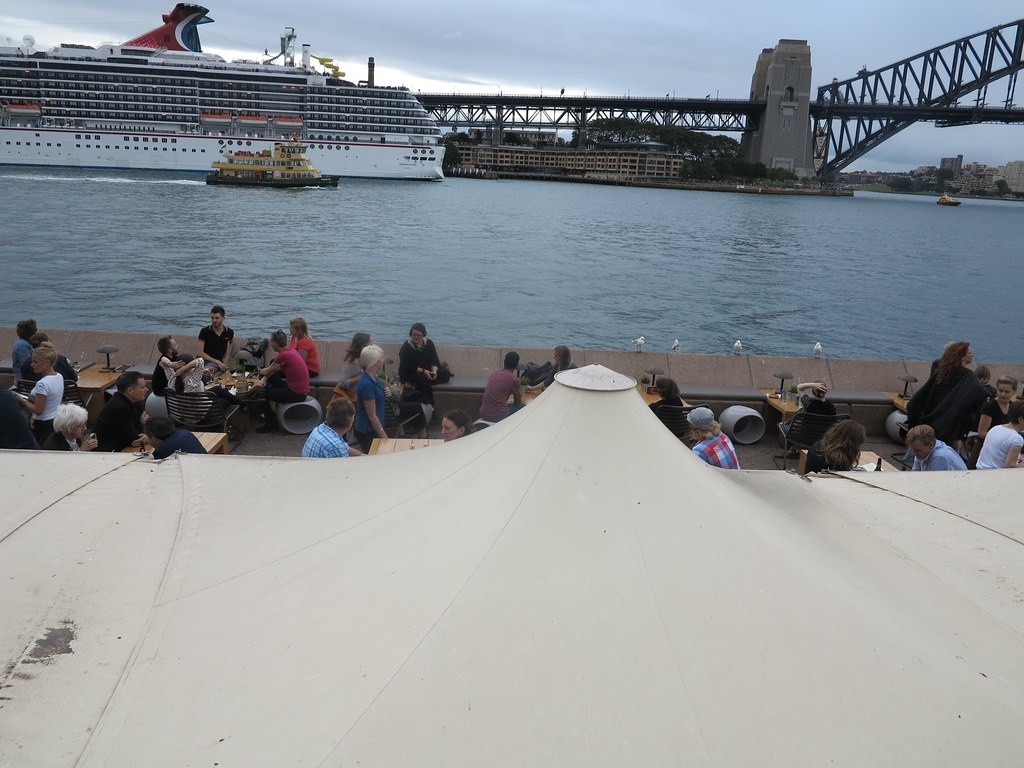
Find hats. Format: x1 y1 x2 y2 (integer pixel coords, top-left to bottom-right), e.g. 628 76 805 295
30 333 47 343
686 407 714 430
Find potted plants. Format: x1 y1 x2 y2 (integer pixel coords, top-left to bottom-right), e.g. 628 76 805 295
235 365 245 378
519 376 528 394
787 384 798 401
641 375 650 392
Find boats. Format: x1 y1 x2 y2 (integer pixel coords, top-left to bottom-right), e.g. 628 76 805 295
937 192 962 206
206 133 340 189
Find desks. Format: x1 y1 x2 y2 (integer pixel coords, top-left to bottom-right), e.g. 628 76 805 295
75 362 123 428
881 390 913 413
757 388 802 422
121 432 230 454
505 384 542 405
636 384 690 413
213 370 266 437
367 438 445 456
797 450 901 472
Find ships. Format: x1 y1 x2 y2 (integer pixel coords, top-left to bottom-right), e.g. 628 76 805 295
0 3 447 181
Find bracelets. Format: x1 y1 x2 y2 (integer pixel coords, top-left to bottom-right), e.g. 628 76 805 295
166 360 170 366
377 427 384 434
421 368 426 375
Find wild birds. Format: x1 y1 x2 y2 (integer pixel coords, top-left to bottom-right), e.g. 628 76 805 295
670 338 681 353
814 343 823 358
632 335 645 352
734 340 742 355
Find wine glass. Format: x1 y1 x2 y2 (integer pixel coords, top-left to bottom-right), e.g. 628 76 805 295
73 362 80 378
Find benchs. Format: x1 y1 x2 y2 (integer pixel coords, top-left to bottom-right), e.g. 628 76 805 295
678 383 767 434
308 369 341 414
804 387 895 436
432 376 487 420
0 358 95 395
124 364 222 410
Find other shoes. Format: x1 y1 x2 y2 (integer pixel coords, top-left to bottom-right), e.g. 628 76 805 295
256 420 280 433
396 433 412 438
788 449 801 456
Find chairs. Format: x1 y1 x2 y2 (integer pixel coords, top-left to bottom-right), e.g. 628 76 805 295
163 387 242 453
773 412 851 470
890 399 915 469
16 378 94 409
383 396 421 438
655 404 709 440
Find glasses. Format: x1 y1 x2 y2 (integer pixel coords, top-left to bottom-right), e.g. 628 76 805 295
274 329 282 343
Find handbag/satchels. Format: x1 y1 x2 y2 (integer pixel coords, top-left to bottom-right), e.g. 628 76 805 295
244 336 269 359
326 384 357 410
384 399 400 430
521 361 553 386
430 362 454 385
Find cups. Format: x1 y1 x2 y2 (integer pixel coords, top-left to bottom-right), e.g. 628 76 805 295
214 378 218 384
225 367 230 374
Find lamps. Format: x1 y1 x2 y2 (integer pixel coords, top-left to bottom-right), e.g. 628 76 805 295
773 370 793 394
382 358 393 374
97 346 119 370
231 349 253 374
643 367 664 395
897 374 918 398
515 362 529 377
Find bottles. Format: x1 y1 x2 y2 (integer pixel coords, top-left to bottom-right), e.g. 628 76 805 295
874 458 882 471
139 441 146 453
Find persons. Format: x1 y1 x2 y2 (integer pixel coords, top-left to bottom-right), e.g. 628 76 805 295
805 420 866 476
255 329 310 433
0 390 41 450
357 345 391 454
92 371 150 452
648 377 684 416
788 380 836 456
398 324 440 407
978 374 1018 440
12 342 64 446
145 416 209 459
196 307 233 381
12 319 77 386
524 345 577 396
441 409 476 443
152 336 247 429
906 425 968 471
287 318 320 378
44 403 98 451
337 332 375 410
301 397 368 458
686 407 740 469
906 340 997 442
479 352 525 422
975 400 1024 470
